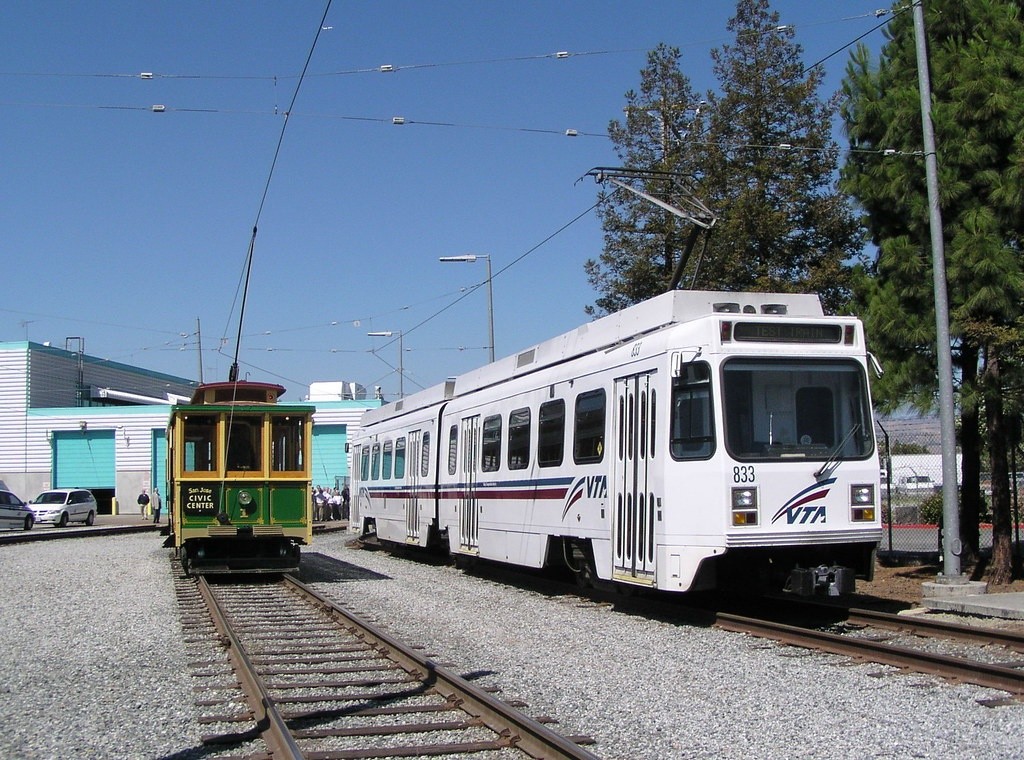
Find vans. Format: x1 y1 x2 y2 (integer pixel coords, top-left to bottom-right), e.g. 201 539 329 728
0 490 36 531
28 488 98 528
898 476 935 498
881 476 896 499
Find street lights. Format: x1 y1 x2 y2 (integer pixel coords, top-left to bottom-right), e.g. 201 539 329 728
365 329 404 398
438 253 495 364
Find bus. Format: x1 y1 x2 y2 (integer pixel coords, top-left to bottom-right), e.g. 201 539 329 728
162 380 317 579
345 288 884 624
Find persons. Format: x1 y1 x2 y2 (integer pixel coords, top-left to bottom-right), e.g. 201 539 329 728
152 488 161 523
312 485 349 521
138 490 150 520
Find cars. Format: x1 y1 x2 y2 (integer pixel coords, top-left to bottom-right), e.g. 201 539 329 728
980 473 1024 493
